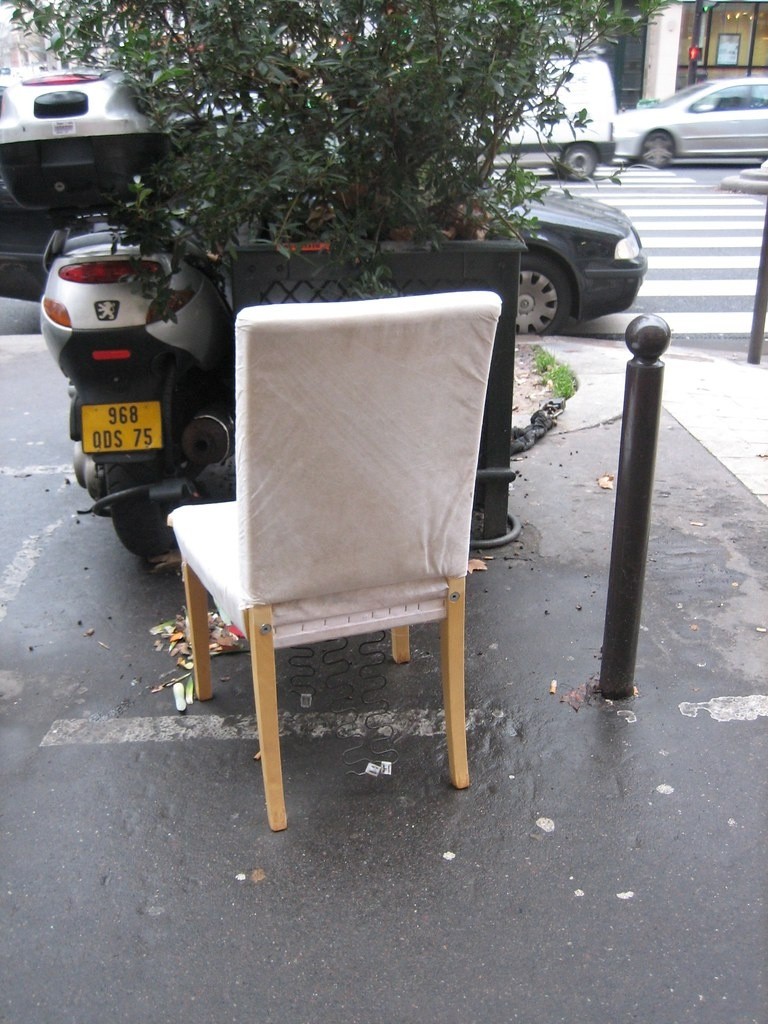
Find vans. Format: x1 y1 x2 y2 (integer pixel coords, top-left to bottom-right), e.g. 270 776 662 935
448 58 619 184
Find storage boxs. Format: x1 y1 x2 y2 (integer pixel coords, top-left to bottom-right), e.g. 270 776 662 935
1 68 167 211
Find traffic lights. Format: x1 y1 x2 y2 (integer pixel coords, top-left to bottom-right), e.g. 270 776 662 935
688 47 702 61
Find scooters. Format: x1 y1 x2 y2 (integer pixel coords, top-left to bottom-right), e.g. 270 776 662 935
0 68 235 559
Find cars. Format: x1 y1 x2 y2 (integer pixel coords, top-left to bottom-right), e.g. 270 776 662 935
0 65 648 337
614 75 768 169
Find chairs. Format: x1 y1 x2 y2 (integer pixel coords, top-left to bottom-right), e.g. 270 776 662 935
163 289 497 833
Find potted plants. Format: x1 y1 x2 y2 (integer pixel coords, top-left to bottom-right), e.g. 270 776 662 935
0 0 691 543
